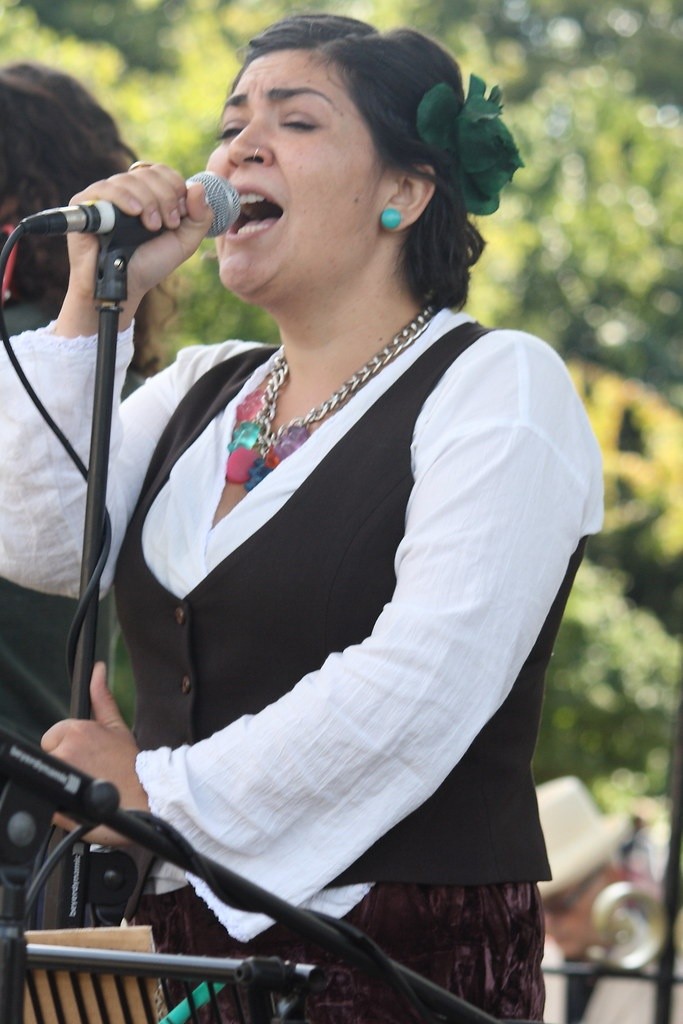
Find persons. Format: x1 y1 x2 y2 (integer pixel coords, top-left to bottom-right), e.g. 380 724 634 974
0 63 180 866
0 13 604 1024
531 776 683 1024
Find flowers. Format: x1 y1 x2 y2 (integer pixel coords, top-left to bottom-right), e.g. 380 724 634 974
416 75 526 215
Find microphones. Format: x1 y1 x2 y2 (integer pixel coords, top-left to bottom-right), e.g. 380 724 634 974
23 170 240 237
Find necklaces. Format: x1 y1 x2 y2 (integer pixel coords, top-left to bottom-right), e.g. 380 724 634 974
225 304 442 489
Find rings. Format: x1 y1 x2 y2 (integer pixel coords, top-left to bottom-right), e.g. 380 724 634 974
126 160 152 172
